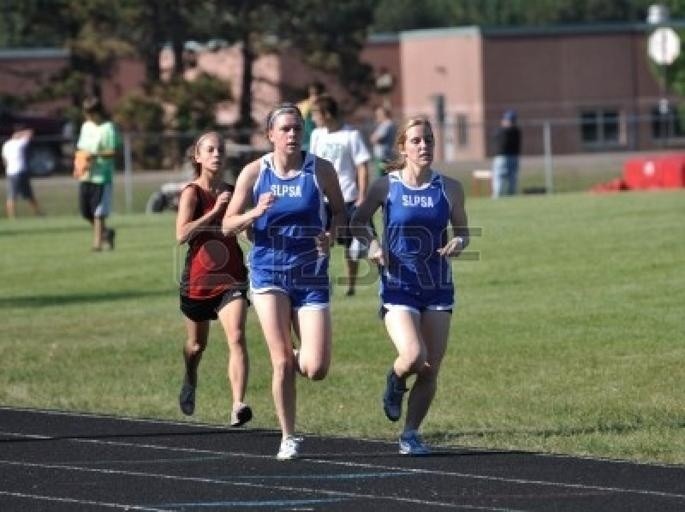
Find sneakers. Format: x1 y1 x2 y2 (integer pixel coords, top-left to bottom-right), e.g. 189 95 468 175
179 384 195 415
383 368 409 420
398 433 431 455
277 434 303 461
230 405 252 426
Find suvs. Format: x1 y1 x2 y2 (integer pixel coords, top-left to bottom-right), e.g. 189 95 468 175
146 142 273 213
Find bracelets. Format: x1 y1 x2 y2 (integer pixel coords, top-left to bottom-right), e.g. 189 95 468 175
453 234 466 248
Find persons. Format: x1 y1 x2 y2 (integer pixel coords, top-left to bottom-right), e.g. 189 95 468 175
347 114 469 454
176 131 253 428
2 128 44 219
221 102 349 462
372 103 395 175
72 94 120 253
292 82 328 149
487 109 524 197
308 96 371 294
